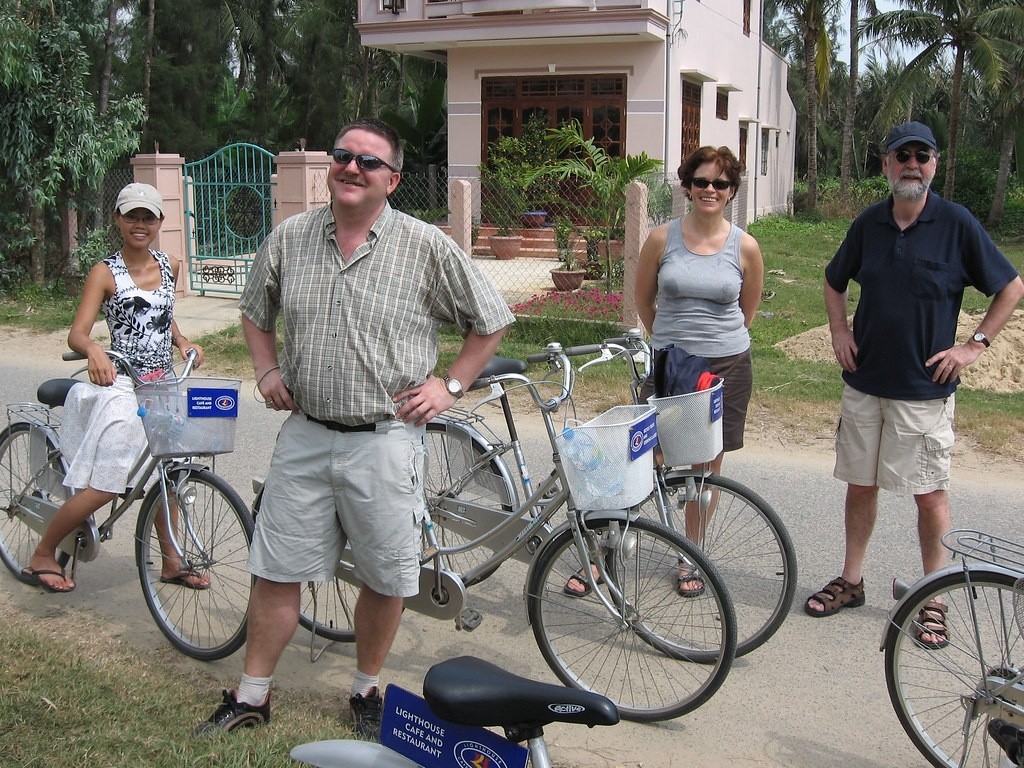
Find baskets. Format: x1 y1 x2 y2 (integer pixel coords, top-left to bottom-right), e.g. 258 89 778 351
133 377 242 457
646 378 724 467
554 404 658 510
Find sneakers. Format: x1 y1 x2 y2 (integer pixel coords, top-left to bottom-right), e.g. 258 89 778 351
350 686 382 742
192 689 271 739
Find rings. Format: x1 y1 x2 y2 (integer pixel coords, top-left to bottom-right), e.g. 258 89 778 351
948 364 953 367
269 400 273 403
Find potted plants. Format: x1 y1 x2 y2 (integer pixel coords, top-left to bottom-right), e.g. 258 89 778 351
504 114 564 230
474 136 533 262
577 228 607 280
553 214 586 291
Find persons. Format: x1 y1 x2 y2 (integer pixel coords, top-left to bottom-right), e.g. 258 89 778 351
563 147 764 596
805 120 1024 650
29 181 212 590
195 118 517 734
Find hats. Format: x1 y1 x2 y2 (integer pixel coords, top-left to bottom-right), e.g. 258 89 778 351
114 183 165 219
884 122 936 154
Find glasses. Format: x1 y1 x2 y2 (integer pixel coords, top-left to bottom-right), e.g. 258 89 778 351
692 177 732 190
895 150 933 163
121 210 158 225
332 149 396 171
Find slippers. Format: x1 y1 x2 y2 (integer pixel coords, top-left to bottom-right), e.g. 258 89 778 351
160 569 210 590
22 566 75 593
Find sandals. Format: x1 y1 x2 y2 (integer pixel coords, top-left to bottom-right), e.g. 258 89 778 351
564 561 606 597
677 556 705 598
917 600 950 649
805 577 866 618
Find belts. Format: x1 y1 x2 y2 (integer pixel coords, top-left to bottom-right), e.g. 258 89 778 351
305 414 377 432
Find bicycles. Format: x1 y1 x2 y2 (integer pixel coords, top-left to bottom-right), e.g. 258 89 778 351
290 655 621 768
421 328 797 664
879 529 1024 768
0 347 258 662
251 342 737 723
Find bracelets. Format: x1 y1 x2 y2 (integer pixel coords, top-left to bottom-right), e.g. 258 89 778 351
173 335 188 345
257 367 280 387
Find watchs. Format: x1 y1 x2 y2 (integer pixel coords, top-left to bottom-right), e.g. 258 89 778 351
973 332 990 348
444 376 463 398
